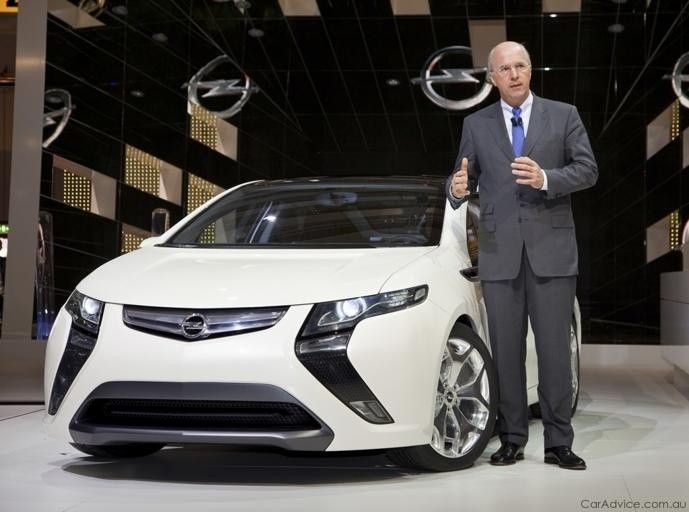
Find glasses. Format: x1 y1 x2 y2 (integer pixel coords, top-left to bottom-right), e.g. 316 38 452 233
494 64 532 76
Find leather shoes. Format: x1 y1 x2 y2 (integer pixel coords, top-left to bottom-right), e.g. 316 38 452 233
543 445 587 469
490 441 525 465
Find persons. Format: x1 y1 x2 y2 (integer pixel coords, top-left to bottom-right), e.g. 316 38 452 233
445 40 600 469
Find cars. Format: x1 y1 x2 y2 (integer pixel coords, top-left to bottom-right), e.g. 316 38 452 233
42 173 582 472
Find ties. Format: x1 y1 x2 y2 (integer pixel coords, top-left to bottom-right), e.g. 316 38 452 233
511 105 527 160
